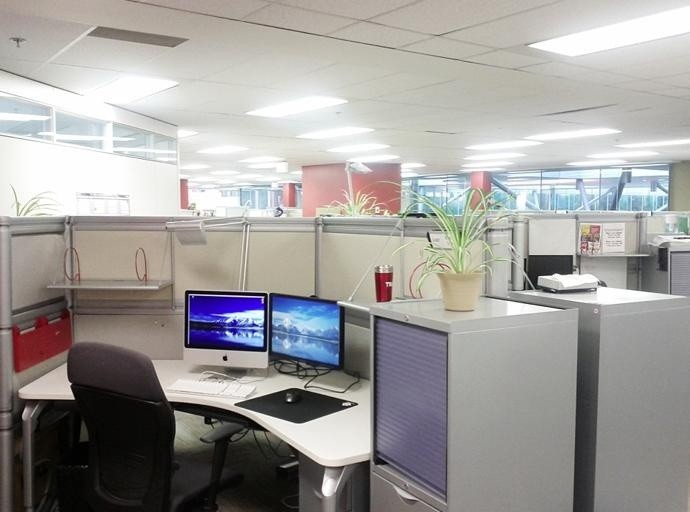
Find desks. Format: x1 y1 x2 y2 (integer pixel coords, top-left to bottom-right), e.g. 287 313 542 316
17 356 373 510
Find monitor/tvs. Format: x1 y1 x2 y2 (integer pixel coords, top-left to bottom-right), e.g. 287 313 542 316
184 289 270 380
270 293 344 371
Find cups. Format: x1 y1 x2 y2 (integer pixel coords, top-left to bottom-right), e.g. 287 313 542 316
374 264 394 302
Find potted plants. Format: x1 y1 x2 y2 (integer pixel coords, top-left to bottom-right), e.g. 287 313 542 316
379 179 535 310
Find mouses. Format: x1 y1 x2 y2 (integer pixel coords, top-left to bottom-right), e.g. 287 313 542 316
285 391 297 403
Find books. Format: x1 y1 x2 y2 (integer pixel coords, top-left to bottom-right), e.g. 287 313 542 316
540 271 600 294
580 224 627 255
165 376 256 400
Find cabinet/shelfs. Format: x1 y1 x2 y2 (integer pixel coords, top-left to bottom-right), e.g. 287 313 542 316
368 295 579 512
512 288 688 511
641 234 689 295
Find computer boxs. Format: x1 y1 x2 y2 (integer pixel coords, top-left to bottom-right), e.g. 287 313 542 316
299 453 370 511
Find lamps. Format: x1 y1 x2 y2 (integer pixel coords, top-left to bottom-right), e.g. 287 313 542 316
163 214 251 293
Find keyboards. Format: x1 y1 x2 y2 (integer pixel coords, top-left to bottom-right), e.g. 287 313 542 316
168 378 256 399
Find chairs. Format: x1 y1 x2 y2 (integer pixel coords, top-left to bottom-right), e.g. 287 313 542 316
67 343 243 512
